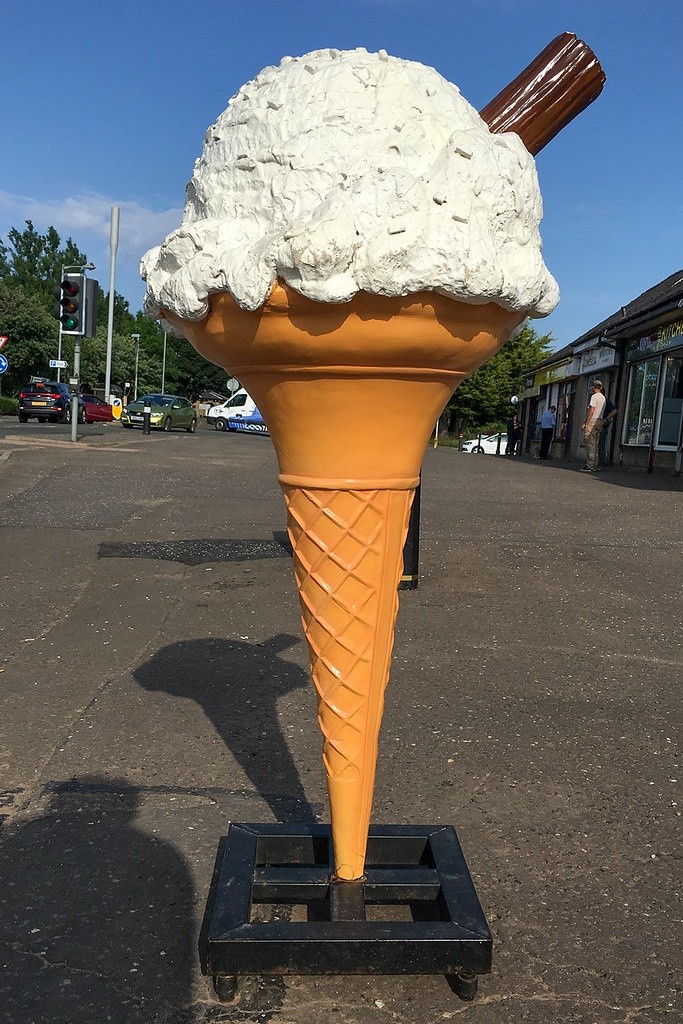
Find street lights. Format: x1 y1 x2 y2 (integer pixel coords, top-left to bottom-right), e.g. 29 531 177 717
636 368 647 442
57 265 96 383
131 334 140 401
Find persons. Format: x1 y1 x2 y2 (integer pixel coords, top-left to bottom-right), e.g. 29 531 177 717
505 412 522 455
578 380 618 472
539 406 557 460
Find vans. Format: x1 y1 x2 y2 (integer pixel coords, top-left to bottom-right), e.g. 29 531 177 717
206 388 269 433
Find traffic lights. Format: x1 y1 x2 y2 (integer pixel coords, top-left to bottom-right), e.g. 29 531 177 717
61 272 87 335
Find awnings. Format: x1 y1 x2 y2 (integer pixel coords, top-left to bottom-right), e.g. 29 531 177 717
606 297 683 340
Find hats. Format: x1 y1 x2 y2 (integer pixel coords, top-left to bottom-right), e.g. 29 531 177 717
592 380 603 387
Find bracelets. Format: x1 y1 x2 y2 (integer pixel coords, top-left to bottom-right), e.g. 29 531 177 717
606 417 609 422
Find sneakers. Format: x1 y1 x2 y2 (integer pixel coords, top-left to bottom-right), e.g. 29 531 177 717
577 465 601 472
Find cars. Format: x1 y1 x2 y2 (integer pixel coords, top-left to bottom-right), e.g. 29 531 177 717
630 433 650 445
80 394 116 423
120 394 197 432
462 432 517 456
17 381 86 425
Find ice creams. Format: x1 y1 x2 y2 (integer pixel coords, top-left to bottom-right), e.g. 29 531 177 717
140 32 606 878
